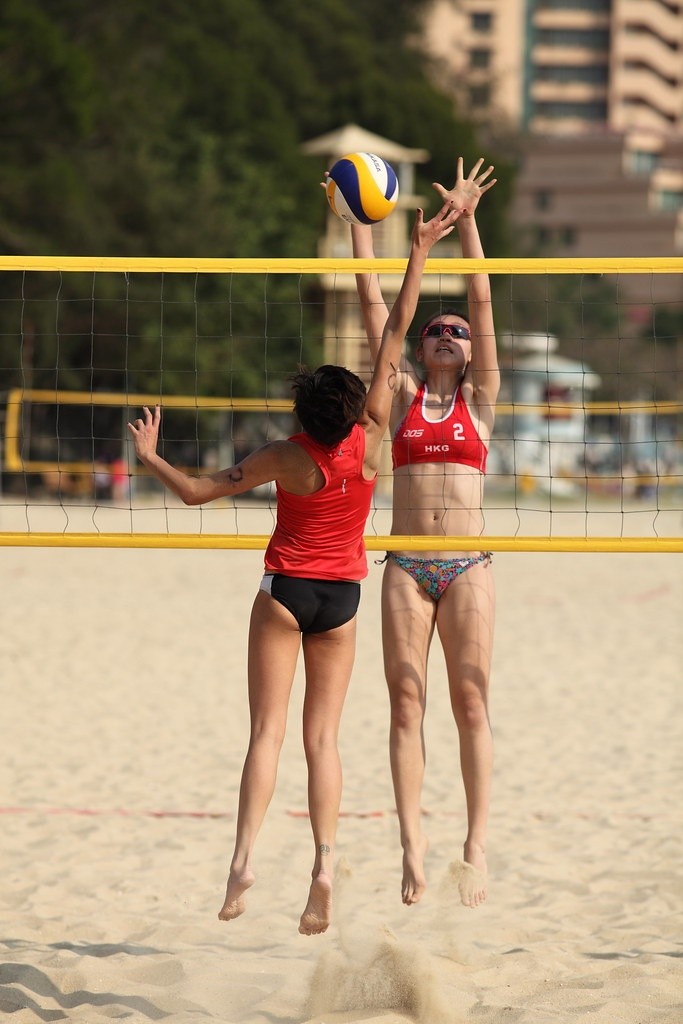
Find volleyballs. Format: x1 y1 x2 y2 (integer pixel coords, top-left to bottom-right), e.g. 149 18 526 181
326 151 399 225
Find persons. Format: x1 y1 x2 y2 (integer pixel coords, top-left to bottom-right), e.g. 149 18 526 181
127 197 468 936
92 457 124 500
320 156 501 907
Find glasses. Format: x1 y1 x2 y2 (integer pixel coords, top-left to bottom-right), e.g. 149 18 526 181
421 322 470 344
